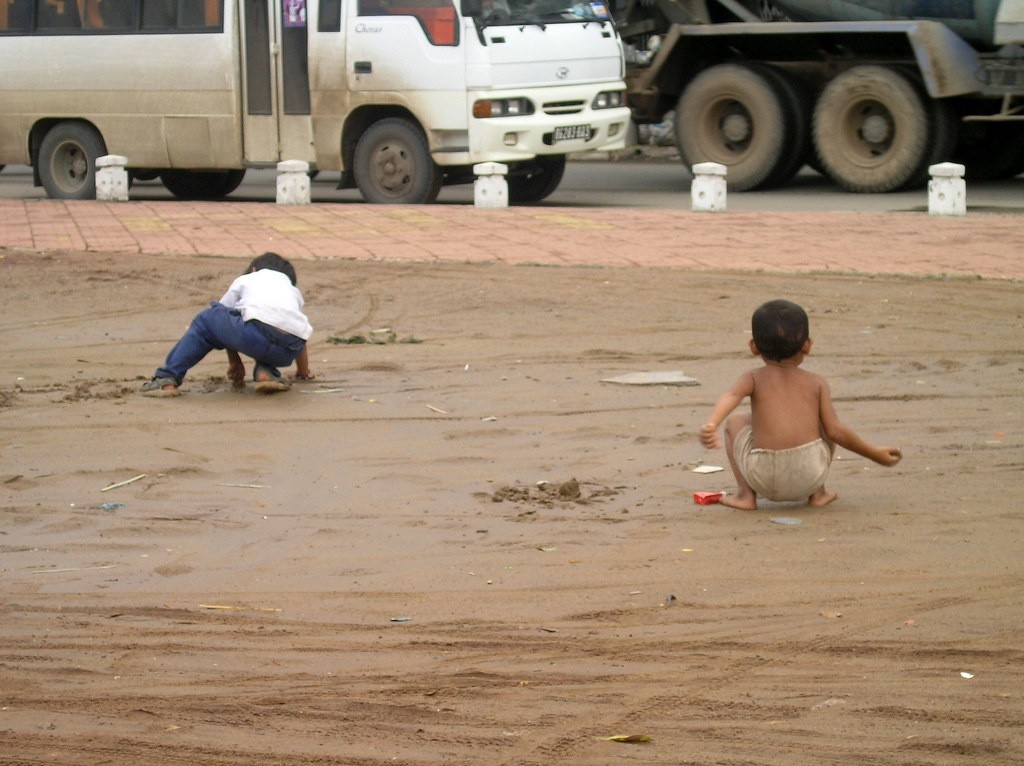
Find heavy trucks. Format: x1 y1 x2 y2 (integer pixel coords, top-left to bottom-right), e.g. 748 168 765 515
606 2 1024 194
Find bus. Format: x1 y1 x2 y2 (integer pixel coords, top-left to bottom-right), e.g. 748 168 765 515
0 0 630 209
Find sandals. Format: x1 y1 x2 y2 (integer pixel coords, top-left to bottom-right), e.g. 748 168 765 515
138 376 180 398
253 363 292 391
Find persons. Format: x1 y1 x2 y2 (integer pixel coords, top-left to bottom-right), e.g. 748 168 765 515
136 251 316 399
696 298 903 511
